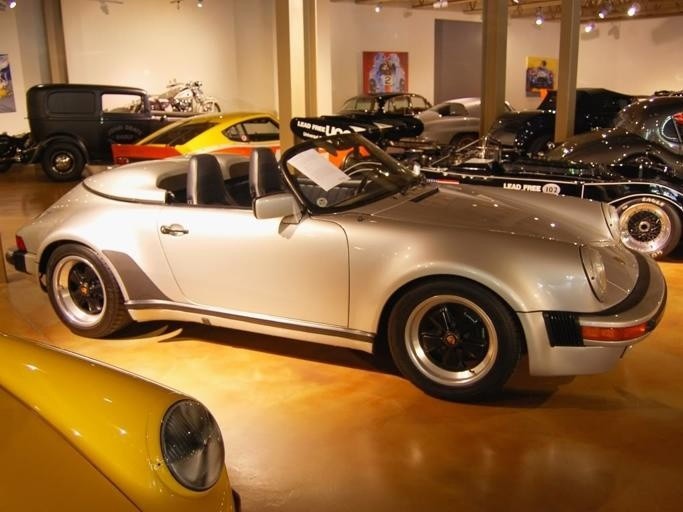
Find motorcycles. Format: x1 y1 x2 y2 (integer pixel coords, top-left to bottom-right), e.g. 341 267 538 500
0 116 32 173
130 78 221 113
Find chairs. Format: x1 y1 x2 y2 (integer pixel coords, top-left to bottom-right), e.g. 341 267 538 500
246 145 286 197
185 154 239 205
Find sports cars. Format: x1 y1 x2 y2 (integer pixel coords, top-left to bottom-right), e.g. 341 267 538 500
111 112 306 165
389 95 516 152
3 130 667 401
284 112 683 261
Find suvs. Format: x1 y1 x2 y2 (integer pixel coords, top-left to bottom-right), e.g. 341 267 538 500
15 83 222 181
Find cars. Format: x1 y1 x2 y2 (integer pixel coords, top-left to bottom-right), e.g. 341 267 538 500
481 87 645 159
0 335 239 512
545 90 683 199
337 92 433 119
